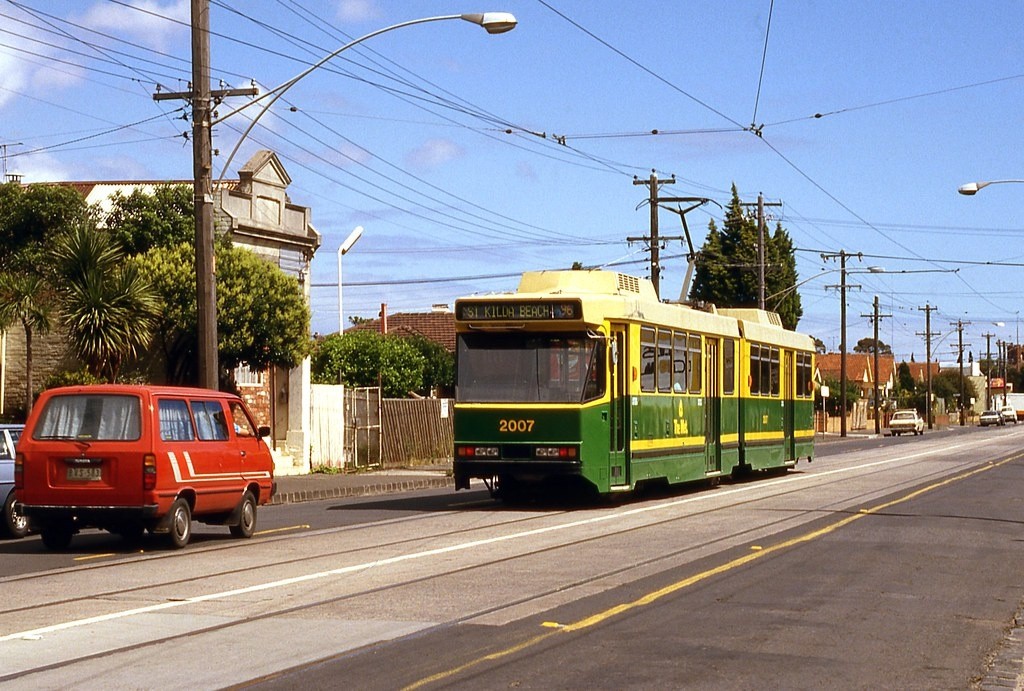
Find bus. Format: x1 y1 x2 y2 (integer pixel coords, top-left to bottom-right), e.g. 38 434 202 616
453 270 816 508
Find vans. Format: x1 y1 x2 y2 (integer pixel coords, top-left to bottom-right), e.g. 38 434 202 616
16 385 277 551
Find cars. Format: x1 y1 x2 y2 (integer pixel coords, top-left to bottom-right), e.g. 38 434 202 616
0 424 40 538
889 408 924 437
979 410 1004 427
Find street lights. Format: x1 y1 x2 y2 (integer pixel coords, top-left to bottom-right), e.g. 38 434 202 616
959 321 1005 426
191 12 519 393
337 225 364 337
958 180 1023 195
758 265 886 315
926 329 968 428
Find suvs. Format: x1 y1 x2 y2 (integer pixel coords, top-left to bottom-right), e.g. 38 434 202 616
1001 406 1017 424
